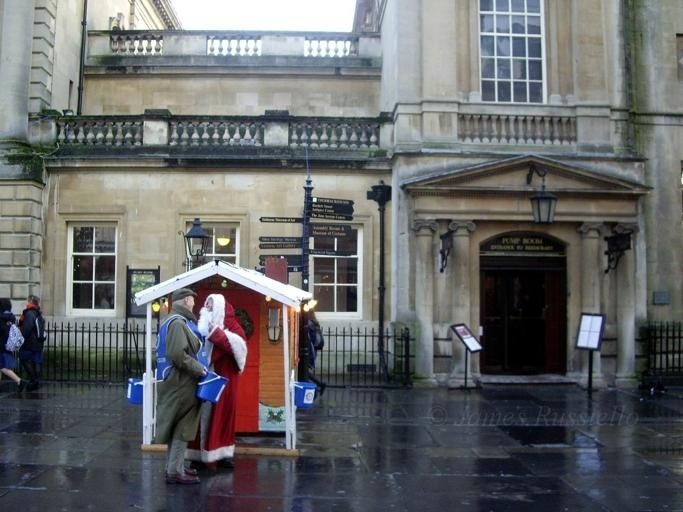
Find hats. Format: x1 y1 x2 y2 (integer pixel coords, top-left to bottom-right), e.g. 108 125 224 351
172 288 197 301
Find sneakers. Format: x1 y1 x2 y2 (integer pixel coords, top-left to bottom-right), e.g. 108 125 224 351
320 384 325 395
217 459 235 467
190 460 207 469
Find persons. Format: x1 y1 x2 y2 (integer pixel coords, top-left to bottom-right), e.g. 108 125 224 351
153 287 209 487
195 293 247 474
298 300 327 400
18 297 49 389
0 297 24 391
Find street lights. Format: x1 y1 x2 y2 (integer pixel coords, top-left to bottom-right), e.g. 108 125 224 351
181 217 214 270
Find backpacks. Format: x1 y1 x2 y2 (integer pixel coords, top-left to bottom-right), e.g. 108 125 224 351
35 316 47 343
5 322 25 351
310 320 324 350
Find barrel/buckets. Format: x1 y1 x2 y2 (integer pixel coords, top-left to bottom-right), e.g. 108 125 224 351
194 370 230 404
293 381 317 409
126 378 144 405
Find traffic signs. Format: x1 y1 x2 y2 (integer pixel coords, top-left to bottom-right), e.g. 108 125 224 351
258 197 354 273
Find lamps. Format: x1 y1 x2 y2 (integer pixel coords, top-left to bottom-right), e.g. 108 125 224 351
176 216 212 263
525 161 559 226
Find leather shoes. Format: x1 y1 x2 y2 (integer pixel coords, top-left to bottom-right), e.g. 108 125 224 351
167 468 200 484
17 380 27 394
